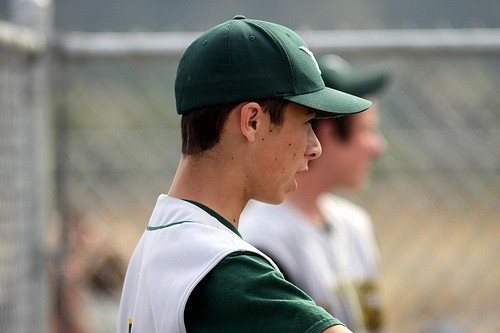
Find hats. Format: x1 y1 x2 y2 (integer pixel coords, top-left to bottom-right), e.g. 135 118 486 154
317 55 397 98
175 15 372 118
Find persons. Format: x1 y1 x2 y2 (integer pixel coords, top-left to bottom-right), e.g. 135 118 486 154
236 52 390 333
116 15 355 333
